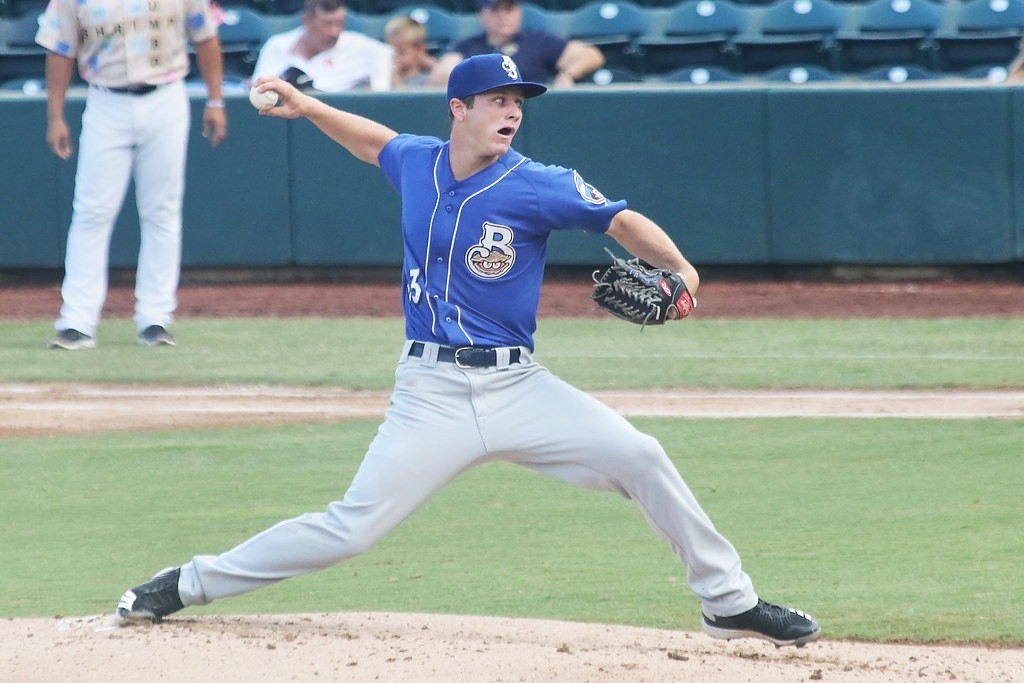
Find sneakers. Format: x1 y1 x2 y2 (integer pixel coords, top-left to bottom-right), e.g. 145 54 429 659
137 324 175 347
49 328 96 350
702 600 823 645
115 564 186 626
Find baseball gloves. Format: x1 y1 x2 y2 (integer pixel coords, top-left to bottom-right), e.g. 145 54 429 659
588 244 698 334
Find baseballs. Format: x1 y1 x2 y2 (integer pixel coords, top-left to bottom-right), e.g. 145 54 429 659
249 82 279 111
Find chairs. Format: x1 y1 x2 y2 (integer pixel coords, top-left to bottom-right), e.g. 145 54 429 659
0 0 1024 92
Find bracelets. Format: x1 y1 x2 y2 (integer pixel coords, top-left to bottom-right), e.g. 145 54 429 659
205 102 225 108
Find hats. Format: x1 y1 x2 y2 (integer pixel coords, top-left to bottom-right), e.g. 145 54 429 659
447 53 547 102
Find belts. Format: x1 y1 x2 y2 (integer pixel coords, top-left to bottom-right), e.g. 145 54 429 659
96 86 156 95
408 343 522 369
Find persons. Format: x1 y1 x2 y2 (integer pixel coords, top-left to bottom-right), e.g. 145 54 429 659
249 2 603 93
35 0 226 351
117 53 822 645
